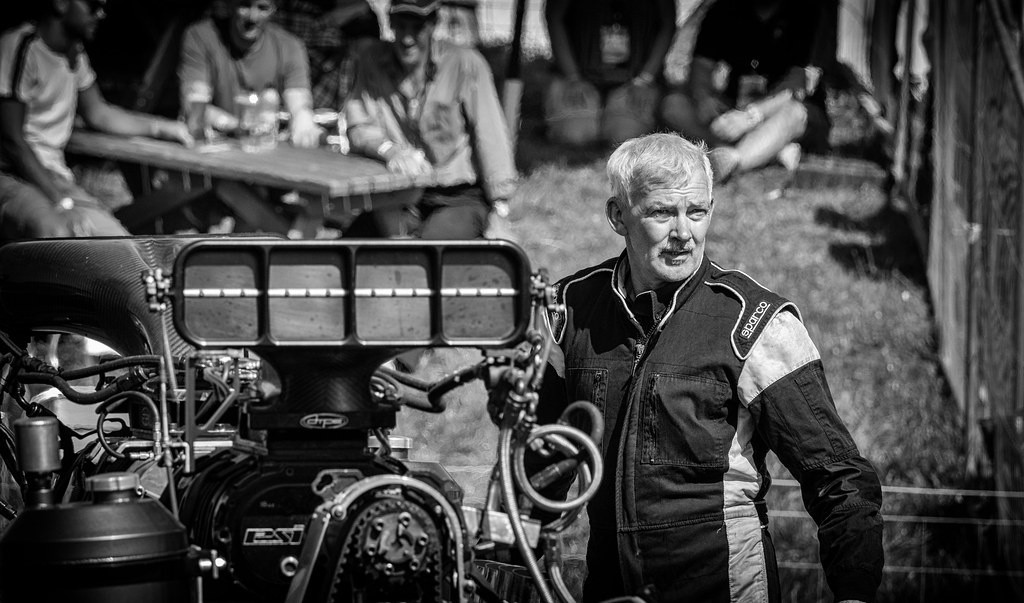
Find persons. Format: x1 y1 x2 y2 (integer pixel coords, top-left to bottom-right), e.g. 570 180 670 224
495 132 886 603
0 0 831 241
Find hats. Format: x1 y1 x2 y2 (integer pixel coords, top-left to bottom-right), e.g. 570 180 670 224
388 0 441 16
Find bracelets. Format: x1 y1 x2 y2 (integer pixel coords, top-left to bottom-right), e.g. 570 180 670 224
54 197 74 213
376 140 394 161
746 103 763 128
490 200 511 219
149 115 164 138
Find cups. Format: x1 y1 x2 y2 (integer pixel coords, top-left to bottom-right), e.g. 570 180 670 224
183 94 279 152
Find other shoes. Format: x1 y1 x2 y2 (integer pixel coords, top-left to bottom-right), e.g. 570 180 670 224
710 147 739 185
777 143 802 177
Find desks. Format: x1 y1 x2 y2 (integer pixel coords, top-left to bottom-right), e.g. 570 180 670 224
70 128 473 239
